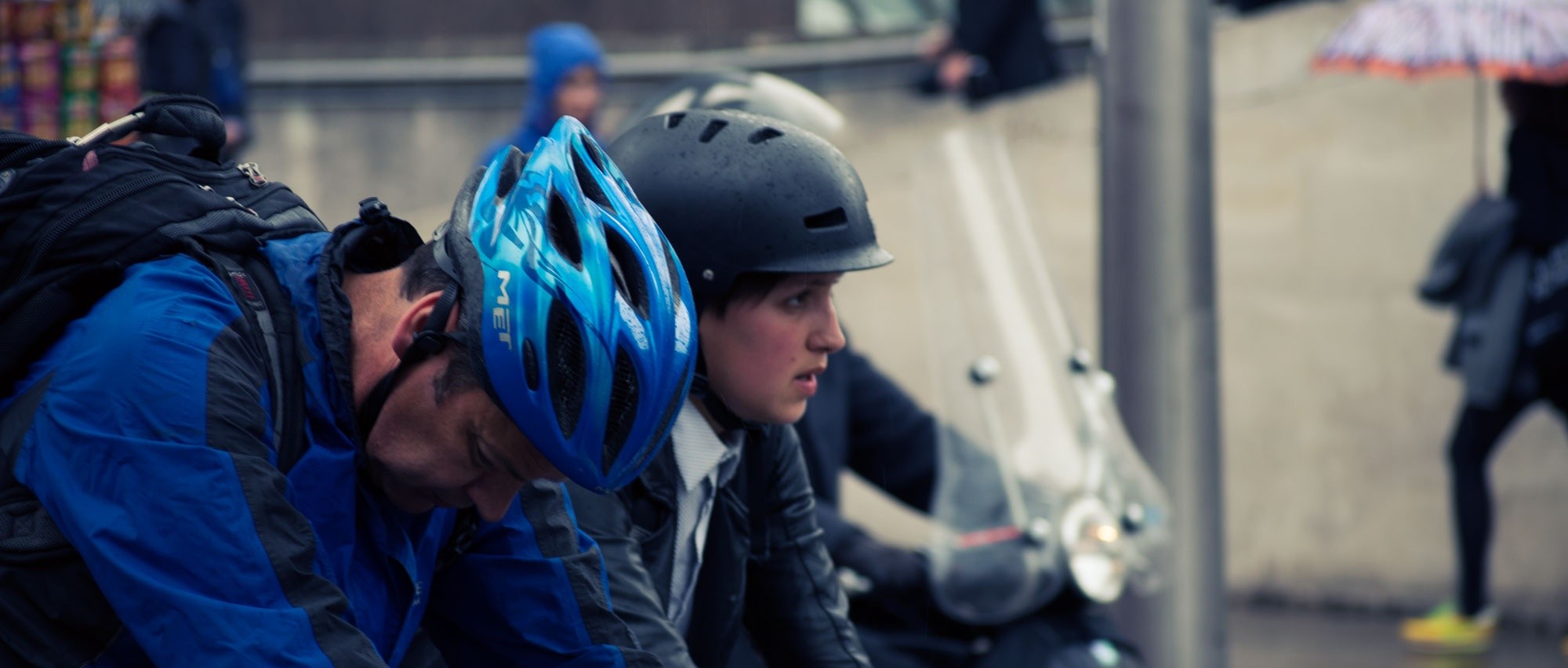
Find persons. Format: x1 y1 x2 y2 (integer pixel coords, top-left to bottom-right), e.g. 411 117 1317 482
1394 73 1567 656
908 0 1093 111
474 20 611 167
0 70 1104 668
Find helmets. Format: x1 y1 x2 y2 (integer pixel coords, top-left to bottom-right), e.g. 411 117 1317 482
604 109 895 276
448 114 701 495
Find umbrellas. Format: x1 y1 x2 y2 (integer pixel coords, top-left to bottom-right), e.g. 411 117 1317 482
1308 2 1567 202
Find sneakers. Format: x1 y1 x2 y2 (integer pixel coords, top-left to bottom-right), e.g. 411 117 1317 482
1397 603 1501 651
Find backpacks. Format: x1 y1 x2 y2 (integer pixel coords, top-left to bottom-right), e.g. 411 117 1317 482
1 94 328 563
